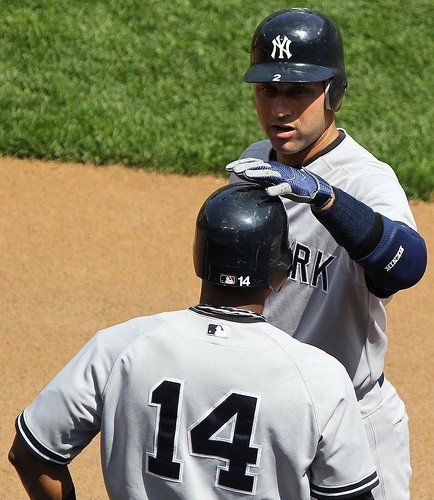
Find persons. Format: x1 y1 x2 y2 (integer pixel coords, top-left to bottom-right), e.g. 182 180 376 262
228 10 427 499
8 180 381 500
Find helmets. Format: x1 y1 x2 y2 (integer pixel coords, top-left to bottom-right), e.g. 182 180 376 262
244 8 347 112
193 182 293 293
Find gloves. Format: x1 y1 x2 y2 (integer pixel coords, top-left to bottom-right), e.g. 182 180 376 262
225 158 333 210
